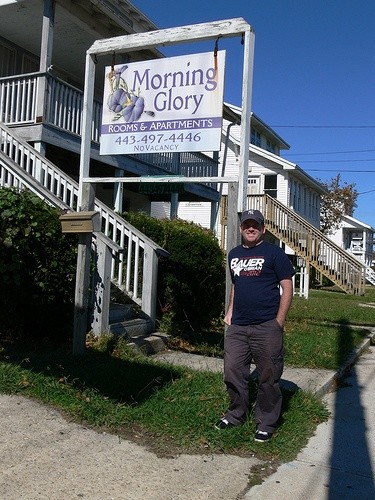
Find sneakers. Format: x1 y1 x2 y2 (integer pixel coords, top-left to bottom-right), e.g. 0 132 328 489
215 417 233 430
254 430 272 443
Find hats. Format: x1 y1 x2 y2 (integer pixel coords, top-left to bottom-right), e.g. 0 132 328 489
241 209 264 227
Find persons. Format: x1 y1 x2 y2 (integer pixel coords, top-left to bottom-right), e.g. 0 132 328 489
213 208 298 441
